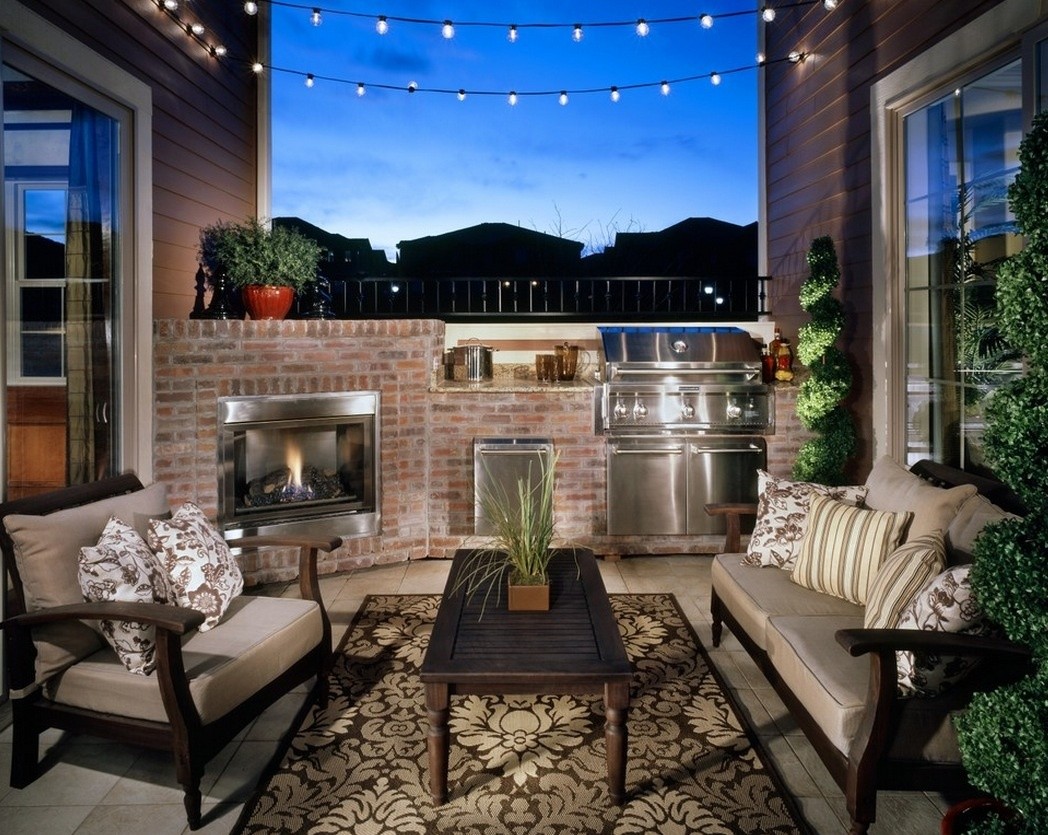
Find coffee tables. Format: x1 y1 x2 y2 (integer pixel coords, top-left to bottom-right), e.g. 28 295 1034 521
420 543 640 807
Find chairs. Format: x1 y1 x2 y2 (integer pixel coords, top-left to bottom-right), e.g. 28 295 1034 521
2 469 343 832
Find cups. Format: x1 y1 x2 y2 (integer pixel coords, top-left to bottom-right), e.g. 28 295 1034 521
556 344 586 380
535 353 560 382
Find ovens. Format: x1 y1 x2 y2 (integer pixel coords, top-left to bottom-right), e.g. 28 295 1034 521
605 435 765 537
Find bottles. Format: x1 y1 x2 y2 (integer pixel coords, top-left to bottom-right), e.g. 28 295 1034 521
762 327 792 382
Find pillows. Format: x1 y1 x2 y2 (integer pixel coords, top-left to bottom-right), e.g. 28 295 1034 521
75 516 185 681
936 492 1028 567
739 466 869 574
857 453 979 549
3 484 171 679
143 501 243 639
789 490 916 609
889 562 990 700
863 529 947 633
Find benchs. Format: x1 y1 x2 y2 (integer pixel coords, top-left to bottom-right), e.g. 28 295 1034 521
705 456 1045 835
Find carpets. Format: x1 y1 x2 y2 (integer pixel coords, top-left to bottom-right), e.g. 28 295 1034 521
223 588 820 835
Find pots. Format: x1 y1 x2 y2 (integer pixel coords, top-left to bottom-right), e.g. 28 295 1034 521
446 339 498 382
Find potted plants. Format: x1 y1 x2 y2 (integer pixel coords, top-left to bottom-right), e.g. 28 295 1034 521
197 212 321 323
443 441 597 624
